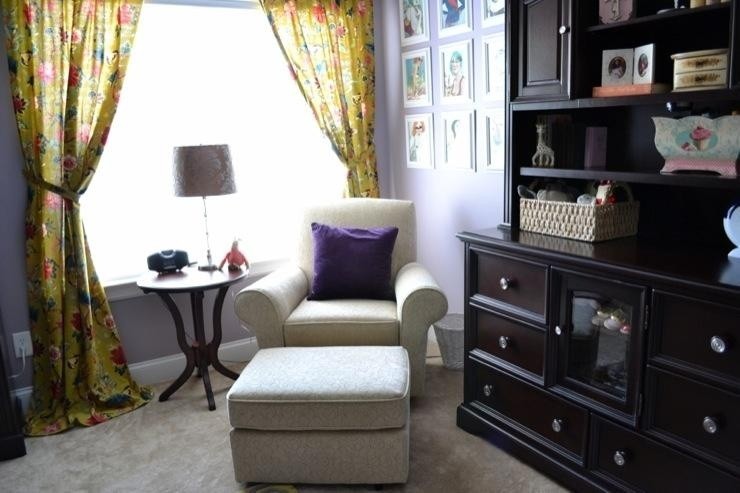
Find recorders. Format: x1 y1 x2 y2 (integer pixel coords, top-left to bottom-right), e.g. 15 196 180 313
148 250 188 272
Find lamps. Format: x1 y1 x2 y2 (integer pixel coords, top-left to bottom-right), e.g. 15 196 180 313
169 140 240 272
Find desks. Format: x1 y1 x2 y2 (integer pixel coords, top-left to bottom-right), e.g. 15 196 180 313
137 254 250 412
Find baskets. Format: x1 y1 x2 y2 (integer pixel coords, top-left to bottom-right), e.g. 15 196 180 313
518 195 642 244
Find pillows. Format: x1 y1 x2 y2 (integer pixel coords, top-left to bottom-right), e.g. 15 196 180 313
309 219 402 302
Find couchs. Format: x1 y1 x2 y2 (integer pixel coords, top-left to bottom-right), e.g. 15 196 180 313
222 343 417 488
229 192 451 400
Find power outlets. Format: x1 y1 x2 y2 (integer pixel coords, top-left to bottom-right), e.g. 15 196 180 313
10 330 35 360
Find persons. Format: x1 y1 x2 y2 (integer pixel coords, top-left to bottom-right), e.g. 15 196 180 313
610 57 625 76
402 0 505 162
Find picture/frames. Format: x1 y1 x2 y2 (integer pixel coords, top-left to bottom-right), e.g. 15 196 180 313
481 31 505 96
436 0 478 36
435 108 477 179
432 34 476 109
484 108 506 171
399 43 434 111
394 0 434 44
399 111 437 174
481 0 509 27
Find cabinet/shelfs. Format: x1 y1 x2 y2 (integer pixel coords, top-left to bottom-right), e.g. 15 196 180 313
449 216 740 493
497 0 740 268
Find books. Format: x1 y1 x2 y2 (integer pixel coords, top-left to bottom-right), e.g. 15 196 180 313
563 123 608 168
